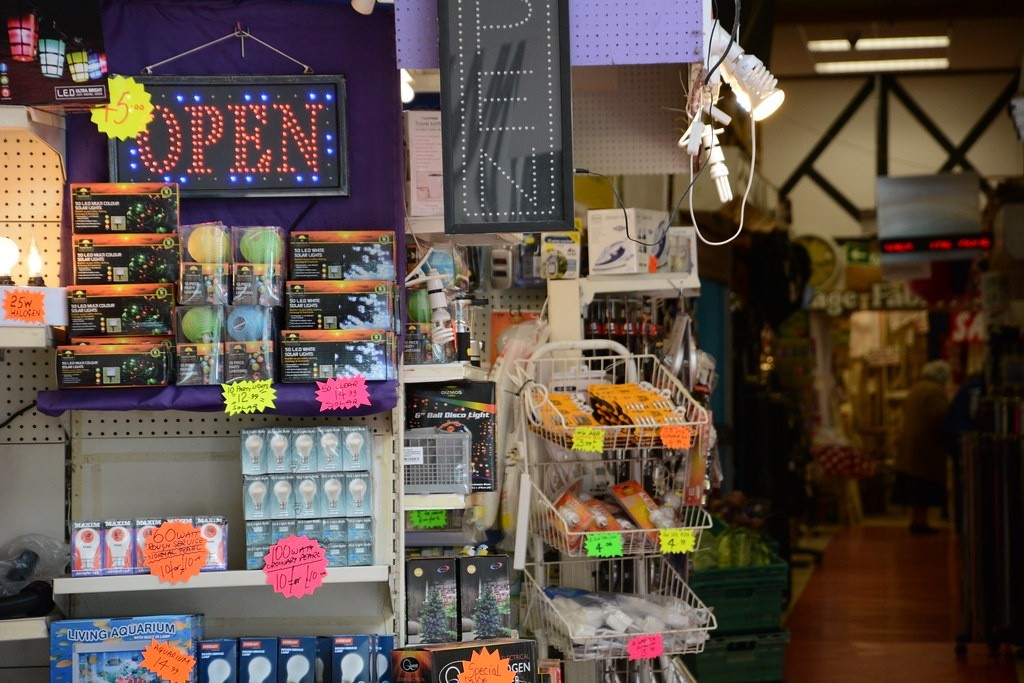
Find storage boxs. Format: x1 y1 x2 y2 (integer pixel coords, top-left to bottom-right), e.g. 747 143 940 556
521 207 699 276
51 612 395 682
54 180 397 385
393 382 538 682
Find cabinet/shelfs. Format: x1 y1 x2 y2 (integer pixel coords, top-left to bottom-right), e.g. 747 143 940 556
0 1 72 682
514 338 718 682
53 0 407 682
405 232 534 683
